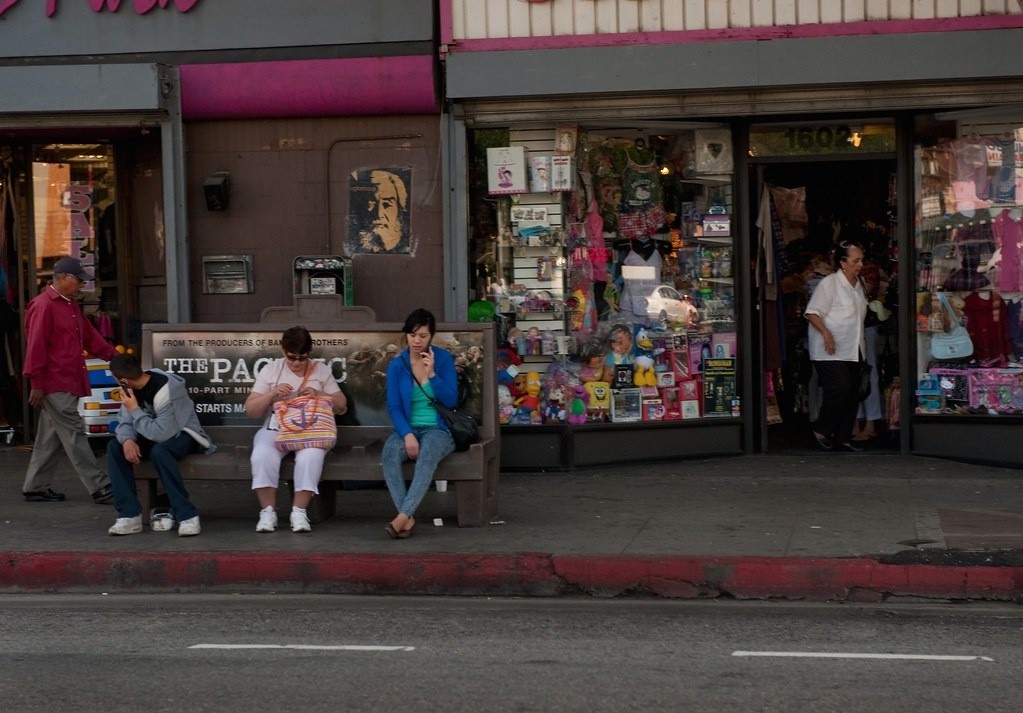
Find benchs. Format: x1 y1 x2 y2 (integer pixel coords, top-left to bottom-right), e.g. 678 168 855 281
132 322 499 528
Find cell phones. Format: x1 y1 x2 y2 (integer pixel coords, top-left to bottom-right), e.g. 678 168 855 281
119 380 131 398
422 337 433 358
267 411 280 431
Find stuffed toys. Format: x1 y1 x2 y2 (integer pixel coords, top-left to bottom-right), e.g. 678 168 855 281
500 324 666 424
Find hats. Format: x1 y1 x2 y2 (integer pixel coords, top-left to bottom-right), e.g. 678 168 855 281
53 257 96 282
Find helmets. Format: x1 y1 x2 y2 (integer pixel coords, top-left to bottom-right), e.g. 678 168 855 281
387 344 397 353
455 357 468 368
360 345 369 352
468 346 480 356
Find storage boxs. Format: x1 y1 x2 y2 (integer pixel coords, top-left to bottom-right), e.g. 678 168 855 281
658 388 682 420
655 372 675 388
551 156 577 191
642 399 662 420
659 349 692 382
609 388 642 422
528 157 552 193
678 379 699 400
486 146 529 194
680 401 700 419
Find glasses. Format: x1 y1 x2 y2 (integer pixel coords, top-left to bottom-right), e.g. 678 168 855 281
283 349 310 362
66 273 85 285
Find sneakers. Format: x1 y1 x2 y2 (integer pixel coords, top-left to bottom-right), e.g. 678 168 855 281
289 506 311 533
108 513 143 535
178 516 201 536
255 506 279 532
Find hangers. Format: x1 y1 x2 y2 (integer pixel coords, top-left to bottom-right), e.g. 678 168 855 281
966 122 983 140
1008 201 1023 214
1002 123 1012 138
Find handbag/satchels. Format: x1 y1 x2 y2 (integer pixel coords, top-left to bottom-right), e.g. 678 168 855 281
929 296 974 359
428 398 482 453
766 185 809 279
273 389 337 452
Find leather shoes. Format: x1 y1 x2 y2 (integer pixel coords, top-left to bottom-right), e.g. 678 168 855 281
92 483 114 503
24 488 66 502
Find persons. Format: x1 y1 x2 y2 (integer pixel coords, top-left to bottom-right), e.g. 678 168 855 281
381 310 459 538
21 257 120 504
245 326 347 532
613 230 672 328
106 354 215 535
350 170 410 253
804 239 892 452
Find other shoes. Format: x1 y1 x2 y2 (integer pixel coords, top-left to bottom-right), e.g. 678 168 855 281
842 440 864 450
384 523 400 538
850 430 877 440
397 522 417 539
813 428 834 449
